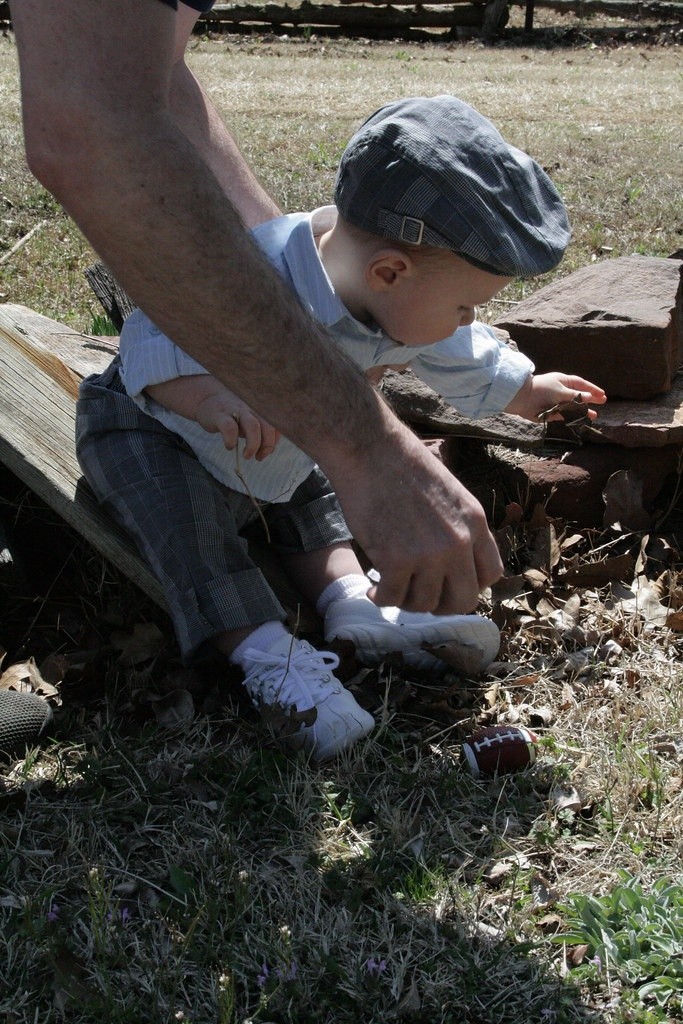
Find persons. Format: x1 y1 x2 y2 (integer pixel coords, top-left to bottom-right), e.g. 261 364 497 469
0 0 509 762
74 96 606 762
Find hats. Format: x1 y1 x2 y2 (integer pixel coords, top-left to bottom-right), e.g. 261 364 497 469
332 94 573 278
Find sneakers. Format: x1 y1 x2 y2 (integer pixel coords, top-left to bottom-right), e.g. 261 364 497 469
242 639 375 759
321 595 501 676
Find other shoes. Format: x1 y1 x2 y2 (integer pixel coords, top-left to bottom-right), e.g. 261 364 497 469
0 691 54 761
